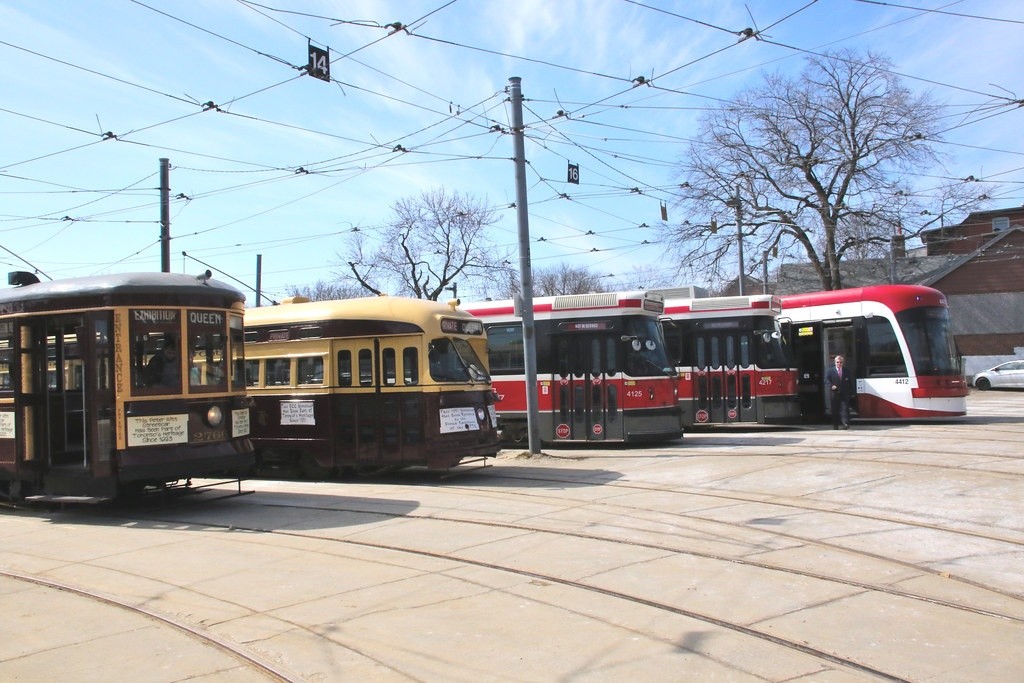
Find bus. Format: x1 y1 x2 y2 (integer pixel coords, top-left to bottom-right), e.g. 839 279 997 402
0 292 506 480
763 284 970 424
1 268 257 511
648 285 807 432
454 290 685 448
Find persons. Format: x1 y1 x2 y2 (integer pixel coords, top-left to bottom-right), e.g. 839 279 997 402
824 356 856 430
144 339 177 386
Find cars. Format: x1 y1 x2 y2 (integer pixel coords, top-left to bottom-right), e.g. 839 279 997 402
971 359 1024 390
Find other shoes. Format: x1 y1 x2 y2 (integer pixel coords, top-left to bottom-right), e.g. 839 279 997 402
833 424 838 430
843 424 849 430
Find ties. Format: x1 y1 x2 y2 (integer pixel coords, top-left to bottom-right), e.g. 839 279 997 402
838 367 842 380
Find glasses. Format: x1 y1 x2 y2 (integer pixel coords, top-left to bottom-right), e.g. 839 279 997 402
837 361 843 364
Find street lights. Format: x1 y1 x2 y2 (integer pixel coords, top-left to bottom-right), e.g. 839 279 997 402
726 183 747 297
